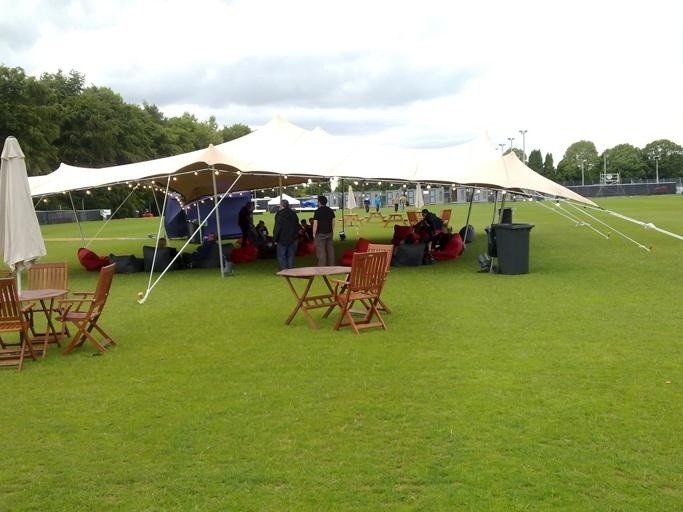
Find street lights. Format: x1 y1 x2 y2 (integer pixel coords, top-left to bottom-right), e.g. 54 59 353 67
496 129 527 166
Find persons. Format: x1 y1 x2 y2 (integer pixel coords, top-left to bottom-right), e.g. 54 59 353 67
363 193 371 214
392 193 400 212
374 193 381 212
311 195 337 267
389 209 452 265
238 200 314 250
398 194 406 211
272 200 302 271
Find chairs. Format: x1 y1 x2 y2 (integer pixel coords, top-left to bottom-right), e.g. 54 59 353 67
2 275 37 372
320 250 389 334
25 261 70 348
54 262 117 355
320 242 394 319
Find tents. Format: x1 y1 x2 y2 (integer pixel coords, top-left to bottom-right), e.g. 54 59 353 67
164 189 252 240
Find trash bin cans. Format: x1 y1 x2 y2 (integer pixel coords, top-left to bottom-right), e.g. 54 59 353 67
499 208 512 224
133 210 140 218
491 224 535 275
185 219 203 244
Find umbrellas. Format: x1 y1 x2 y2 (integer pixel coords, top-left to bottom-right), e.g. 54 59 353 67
0 135 46 348
345 185 358 225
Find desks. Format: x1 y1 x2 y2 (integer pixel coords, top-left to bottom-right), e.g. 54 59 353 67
276 264 352 329
1 288 69 360
